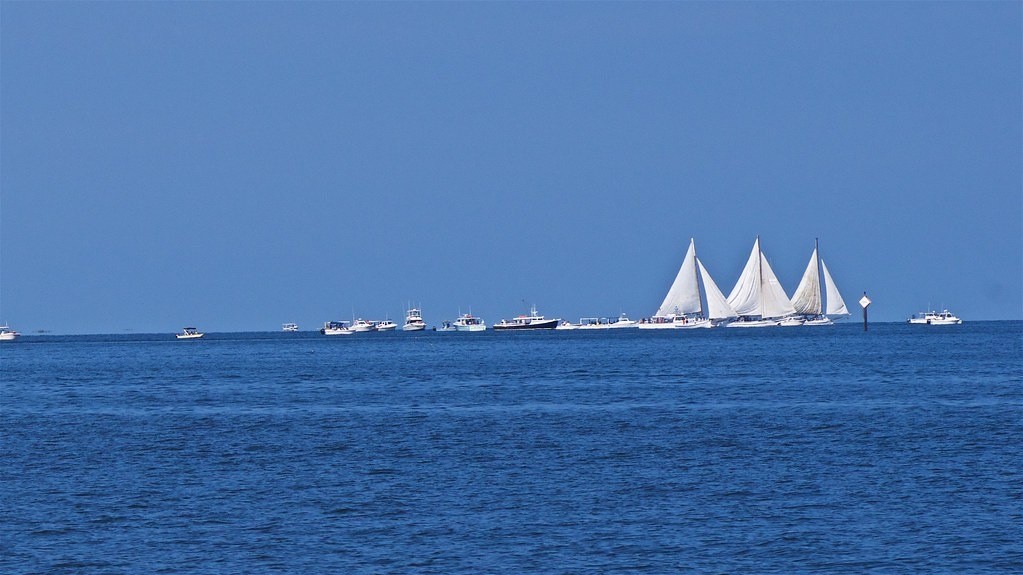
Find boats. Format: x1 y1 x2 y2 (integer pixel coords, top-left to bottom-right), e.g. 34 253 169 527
350 321 380 331
580 315 674 329
437 321 456 331
780 318 802 326
13 331 20 337
324 320 356 335
908 308 963 325
556 320 582 329
492 304 562 331
376 321 397 331
282 323 299 332
175 327 203 339
0 326 16 340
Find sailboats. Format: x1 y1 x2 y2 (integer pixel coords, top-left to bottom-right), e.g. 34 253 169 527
651 238 739 329
452 306 487 332
727 234 797 328
402 301 427 331
791 238 851 325
